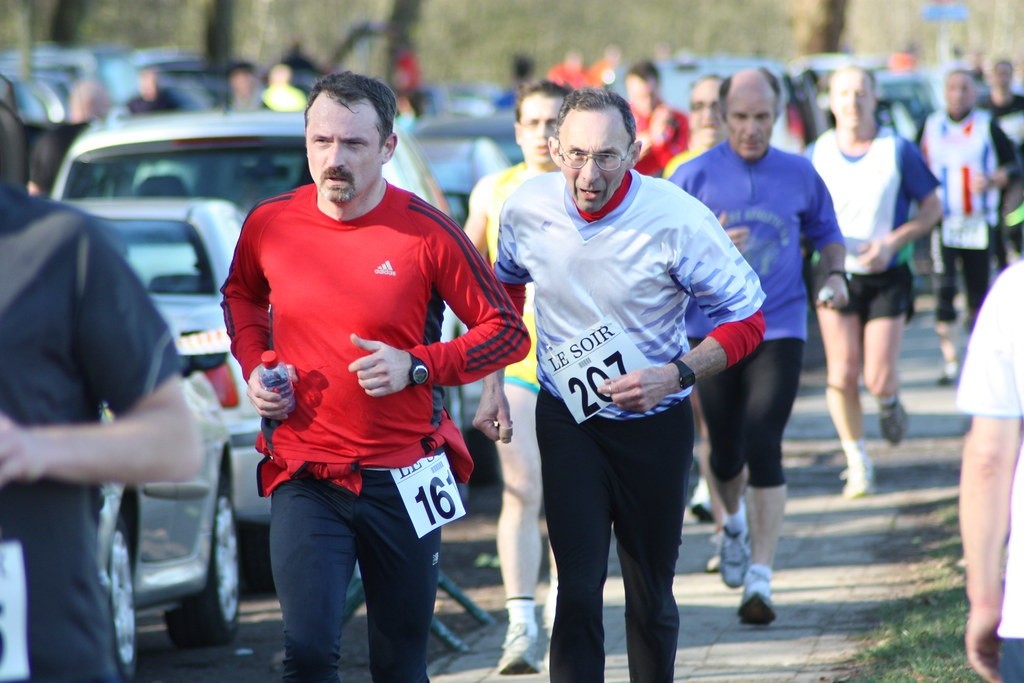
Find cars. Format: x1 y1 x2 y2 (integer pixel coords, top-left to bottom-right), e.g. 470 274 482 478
0 44 947 232
49 105 499 470
59 197 276 574
96 370 242 683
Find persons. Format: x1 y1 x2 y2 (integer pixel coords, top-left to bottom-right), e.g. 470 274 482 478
473 88 765 683
810 69 946 487
0 76 206 683
773 67 836 141
932 61 1024 383
220 70 533 683
622 60 725 177
1 58 310 179
672 67 851 625
467 79 574 673
954 261 1024 683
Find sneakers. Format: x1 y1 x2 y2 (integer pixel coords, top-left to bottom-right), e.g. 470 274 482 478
719 499 750 588
497 622 543 674
879 395 909 444
739 573 777 626
689 477 714 521
841 446 877 498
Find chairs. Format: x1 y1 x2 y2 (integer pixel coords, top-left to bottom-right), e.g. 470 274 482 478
140 176 190 195
153 274 204 293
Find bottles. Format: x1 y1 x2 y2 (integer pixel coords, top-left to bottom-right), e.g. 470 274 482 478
257 350 296 414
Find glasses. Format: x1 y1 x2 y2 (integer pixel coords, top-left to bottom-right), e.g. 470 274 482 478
557 138 632 171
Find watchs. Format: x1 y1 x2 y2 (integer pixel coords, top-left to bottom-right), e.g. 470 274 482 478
408 355 430 385
672 360 696 388
831 269 853 283
